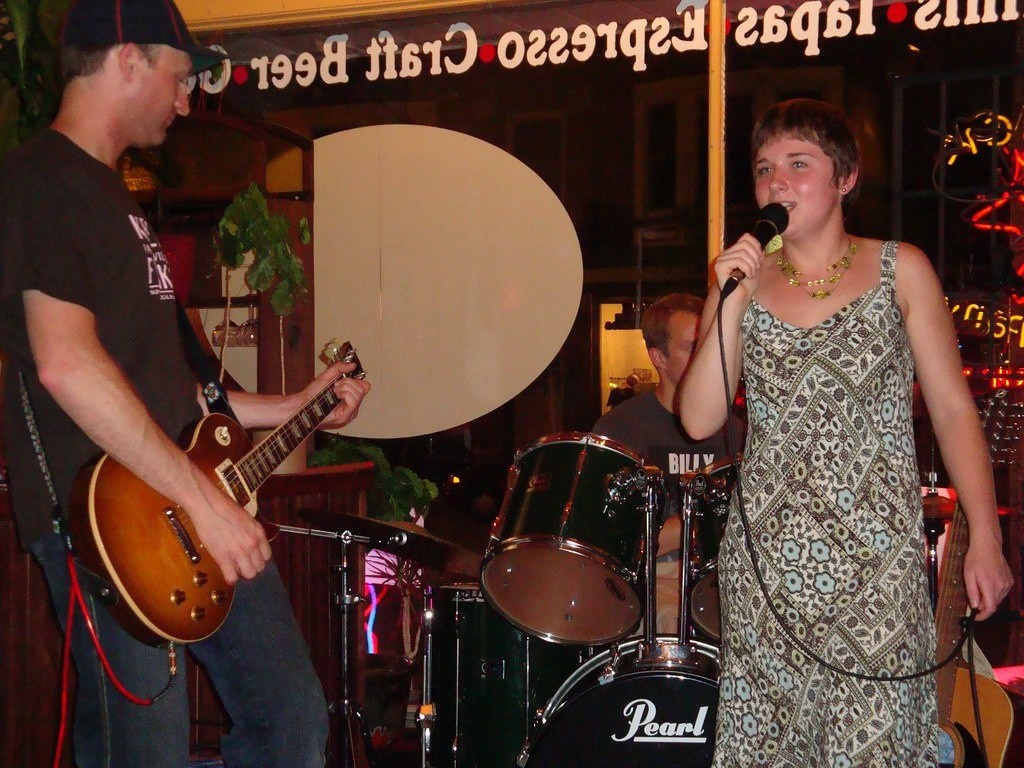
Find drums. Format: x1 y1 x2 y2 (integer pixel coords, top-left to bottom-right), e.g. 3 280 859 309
518 636 724 768
475 428 665 648
686 455 745 646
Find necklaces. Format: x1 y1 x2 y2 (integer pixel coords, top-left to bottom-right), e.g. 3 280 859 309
777 235 856 299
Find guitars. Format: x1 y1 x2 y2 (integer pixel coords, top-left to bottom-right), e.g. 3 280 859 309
62 336 370 651
925 386 1023 767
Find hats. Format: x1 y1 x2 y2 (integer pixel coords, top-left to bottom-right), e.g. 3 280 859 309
66 0 230 74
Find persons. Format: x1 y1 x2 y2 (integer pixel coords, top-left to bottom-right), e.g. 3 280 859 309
594 295 748 637
679 102 1015 768
0 0 370 768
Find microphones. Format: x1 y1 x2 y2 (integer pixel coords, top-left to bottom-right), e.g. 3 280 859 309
718 202 789 299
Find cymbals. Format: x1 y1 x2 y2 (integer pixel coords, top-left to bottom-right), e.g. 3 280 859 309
295 503 467 575
